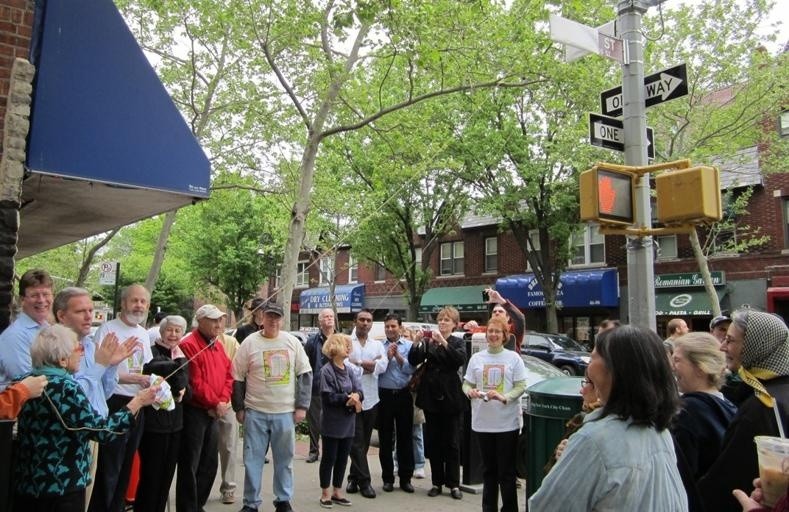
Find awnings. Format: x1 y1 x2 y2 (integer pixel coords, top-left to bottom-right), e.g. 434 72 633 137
420 270 618 311
656 290 725 315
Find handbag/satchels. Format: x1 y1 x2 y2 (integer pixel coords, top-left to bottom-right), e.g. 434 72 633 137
408 364 428 392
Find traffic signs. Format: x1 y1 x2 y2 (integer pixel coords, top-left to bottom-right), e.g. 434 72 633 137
587 110 658 159
597 61 691 119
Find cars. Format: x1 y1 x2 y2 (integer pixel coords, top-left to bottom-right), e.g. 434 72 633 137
221 321 590 482
568 326 600 352
90 304 105 326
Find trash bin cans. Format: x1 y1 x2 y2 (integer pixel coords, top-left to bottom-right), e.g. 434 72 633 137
523 376 587 512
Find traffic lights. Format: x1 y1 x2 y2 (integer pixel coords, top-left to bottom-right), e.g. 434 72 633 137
578 166 634 227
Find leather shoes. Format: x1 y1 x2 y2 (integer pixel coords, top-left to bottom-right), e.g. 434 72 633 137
222 453 462 512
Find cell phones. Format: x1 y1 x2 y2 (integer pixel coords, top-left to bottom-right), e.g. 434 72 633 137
482 291 490 301
423 329 432 338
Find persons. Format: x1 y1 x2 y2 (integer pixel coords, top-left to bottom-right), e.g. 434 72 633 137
299 286 526 510
528 309 789 511
0 268 313 511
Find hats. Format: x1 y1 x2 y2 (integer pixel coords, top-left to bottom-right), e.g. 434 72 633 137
711 316 732 329
195 299 284 322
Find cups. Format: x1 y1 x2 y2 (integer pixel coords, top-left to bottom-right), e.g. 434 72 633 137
753 434 789 507
